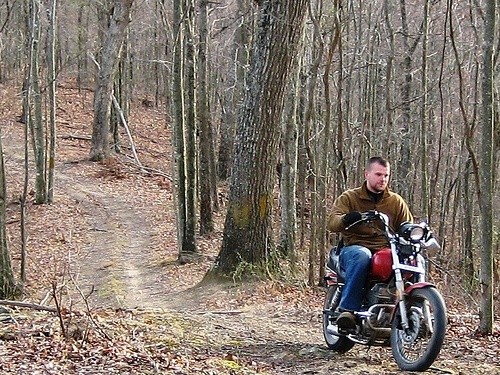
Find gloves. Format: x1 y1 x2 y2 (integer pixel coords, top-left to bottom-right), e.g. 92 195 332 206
344 212 362 226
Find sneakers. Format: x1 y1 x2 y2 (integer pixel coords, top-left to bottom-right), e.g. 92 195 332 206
335 311 355 327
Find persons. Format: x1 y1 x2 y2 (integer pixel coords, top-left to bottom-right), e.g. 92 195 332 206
329 156 414 331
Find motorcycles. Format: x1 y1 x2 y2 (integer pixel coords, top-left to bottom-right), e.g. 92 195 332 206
322 209 447 370
